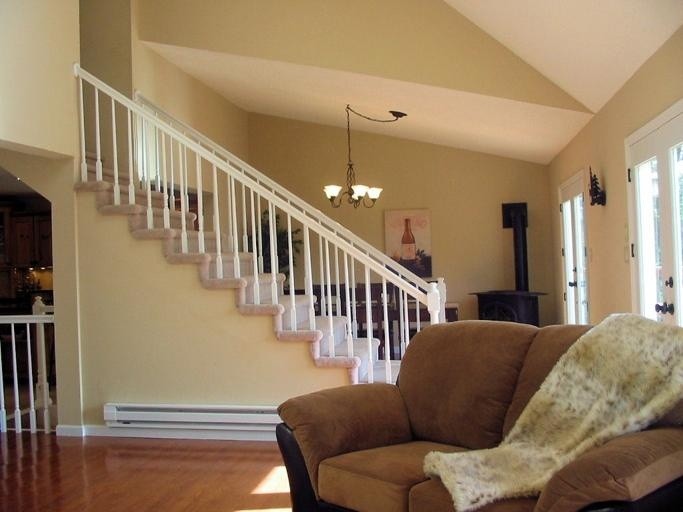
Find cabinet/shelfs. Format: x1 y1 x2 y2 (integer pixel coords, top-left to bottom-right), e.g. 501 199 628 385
9 215 53 269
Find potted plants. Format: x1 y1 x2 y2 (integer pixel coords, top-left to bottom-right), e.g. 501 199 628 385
242 209 304 290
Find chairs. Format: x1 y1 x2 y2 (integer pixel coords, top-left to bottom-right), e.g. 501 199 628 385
313 281 399 360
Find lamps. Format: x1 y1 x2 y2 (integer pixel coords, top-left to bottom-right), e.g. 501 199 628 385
323 105 408 209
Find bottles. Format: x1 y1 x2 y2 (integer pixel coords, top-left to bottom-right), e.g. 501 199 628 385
399 219 416 267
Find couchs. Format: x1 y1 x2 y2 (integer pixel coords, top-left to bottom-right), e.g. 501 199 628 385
274 320 682 510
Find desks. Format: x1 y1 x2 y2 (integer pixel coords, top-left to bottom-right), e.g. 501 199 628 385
313 303 458 361
